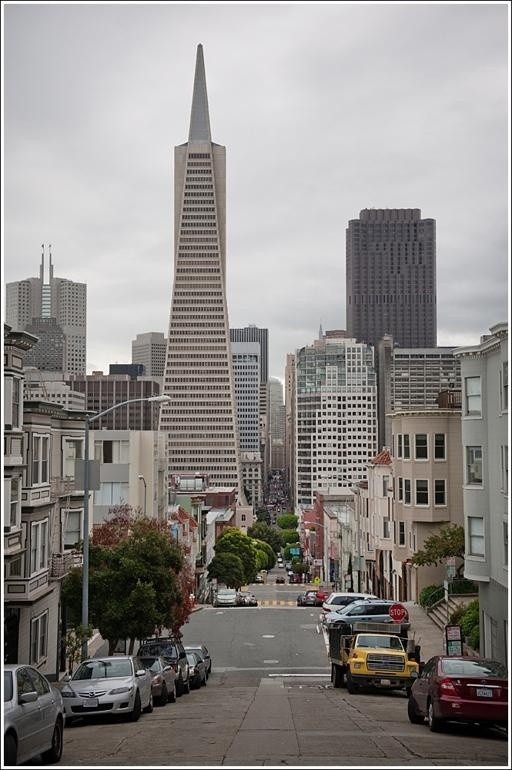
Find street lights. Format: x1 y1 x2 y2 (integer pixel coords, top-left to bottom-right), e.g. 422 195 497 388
138 475 147 521
81 395 172 663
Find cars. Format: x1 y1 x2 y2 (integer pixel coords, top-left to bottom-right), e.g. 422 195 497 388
276 576 285 583
408 655 507 733
255 570 268 583
296 590 331 606
4 664 64 766
62 656 154 722
237 591 258 606
319 593 411 629
285 562 301 583
277 553 284 568
137 640 211 707
217 589 237 606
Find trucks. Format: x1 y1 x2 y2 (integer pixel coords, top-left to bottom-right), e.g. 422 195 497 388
328 622 420 696
